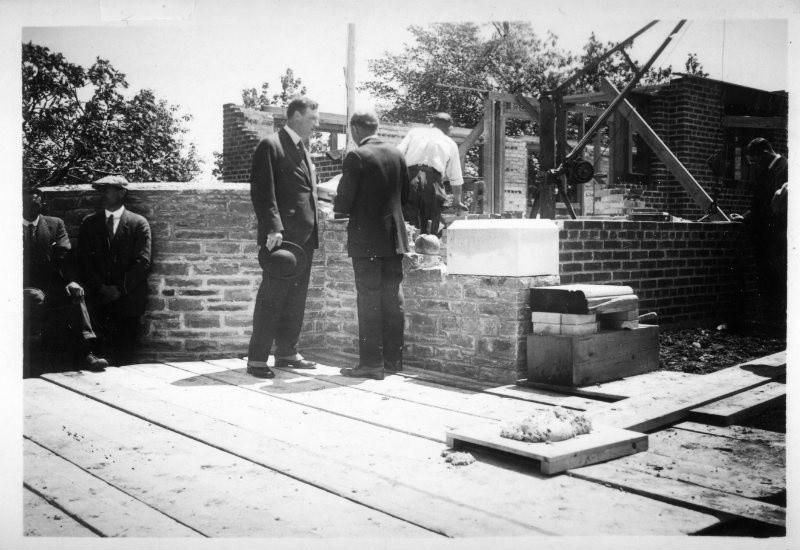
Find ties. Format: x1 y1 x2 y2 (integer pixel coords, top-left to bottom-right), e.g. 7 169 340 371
106 215 114 245
28 224 34 240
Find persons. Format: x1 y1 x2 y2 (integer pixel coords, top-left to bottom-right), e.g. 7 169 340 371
728 137 788 323
23 175 152 381
334 110 409 380
395 112 468 235
248 98 320 375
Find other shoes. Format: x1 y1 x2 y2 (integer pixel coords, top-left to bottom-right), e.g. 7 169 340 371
72 350 109 371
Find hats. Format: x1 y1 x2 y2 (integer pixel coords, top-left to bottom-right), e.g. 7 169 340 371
91 176 130 191
259 240 307 280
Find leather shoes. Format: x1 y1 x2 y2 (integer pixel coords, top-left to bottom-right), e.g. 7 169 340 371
247 364 275 378
341 364 384 381
384 358 402 372
275 355 316 369
